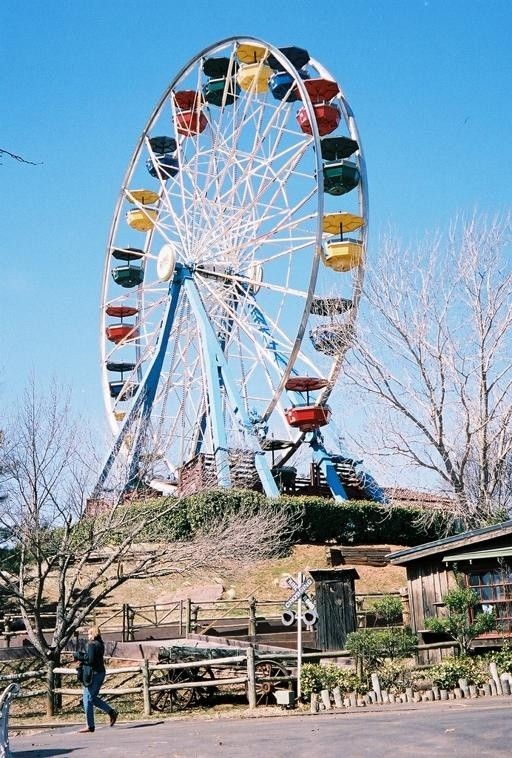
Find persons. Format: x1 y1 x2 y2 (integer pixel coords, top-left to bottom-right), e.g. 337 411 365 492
74 627 119 732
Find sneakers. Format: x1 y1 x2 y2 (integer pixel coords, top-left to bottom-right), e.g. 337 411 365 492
79 726 94 732
109 710 119 726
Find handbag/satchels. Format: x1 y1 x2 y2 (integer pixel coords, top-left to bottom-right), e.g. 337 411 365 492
77 663 93 685
